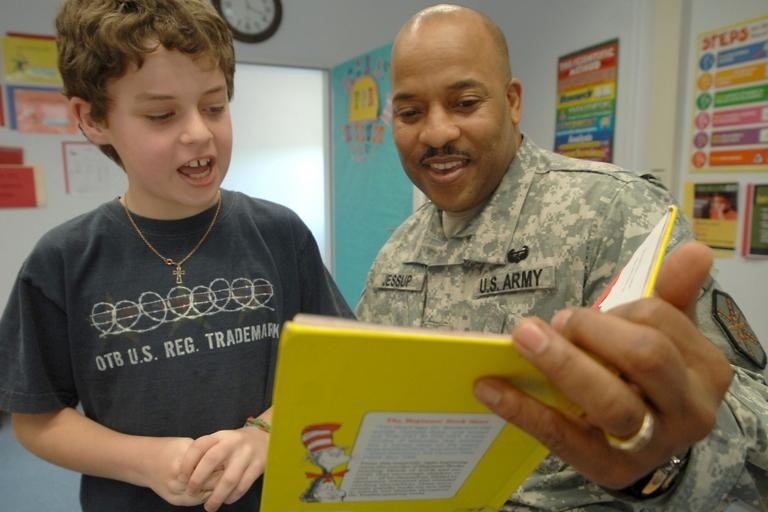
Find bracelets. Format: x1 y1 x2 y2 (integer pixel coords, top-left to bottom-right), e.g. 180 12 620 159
244 415 272 433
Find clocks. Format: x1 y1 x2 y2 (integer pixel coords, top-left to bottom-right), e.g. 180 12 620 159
212 0 283 46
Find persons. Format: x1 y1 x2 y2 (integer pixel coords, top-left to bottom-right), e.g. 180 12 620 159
709 193 737 221
0 0 349 512
352 5 767 512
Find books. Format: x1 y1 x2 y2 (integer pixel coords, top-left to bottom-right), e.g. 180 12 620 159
258 204 678 508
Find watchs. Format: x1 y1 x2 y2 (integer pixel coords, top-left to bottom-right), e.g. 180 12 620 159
632 452 697 498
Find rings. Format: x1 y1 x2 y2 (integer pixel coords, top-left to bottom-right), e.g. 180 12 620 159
607 416 657 454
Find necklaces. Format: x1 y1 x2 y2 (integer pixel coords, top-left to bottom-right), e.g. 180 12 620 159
122 192 224 282
